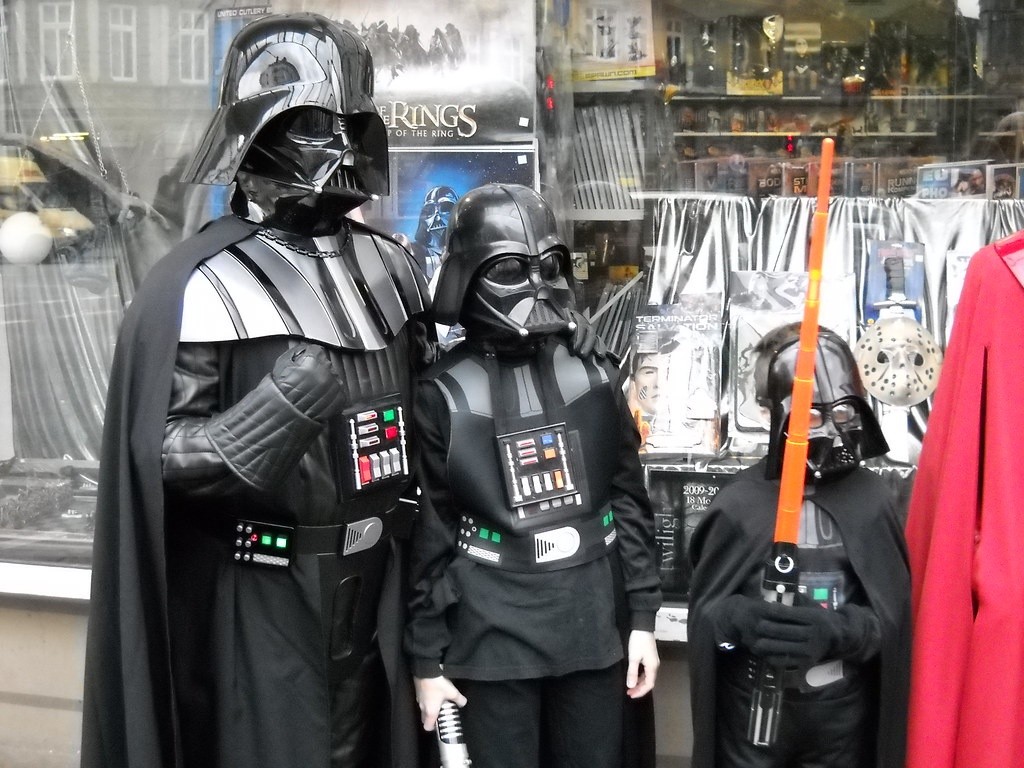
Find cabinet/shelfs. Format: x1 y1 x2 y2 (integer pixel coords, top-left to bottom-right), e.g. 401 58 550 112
671 95 1016 162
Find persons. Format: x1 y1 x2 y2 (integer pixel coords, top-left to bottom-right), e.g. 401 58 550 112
79 13 622 768
906 224 1024 768
687 323 910 768
632 338 683 414
393 185 460 282
403 183 661 768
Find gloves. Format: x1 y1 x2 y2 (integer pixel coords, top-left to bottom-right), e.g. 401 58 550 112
270 343 347 424
711 593 779 664
746 605 867 689
564 308 622 365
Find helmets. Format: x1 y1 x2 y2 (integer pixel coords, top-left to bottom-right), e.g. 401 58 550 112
179 12 390 235
430 182 577 337
764 333 890 480
